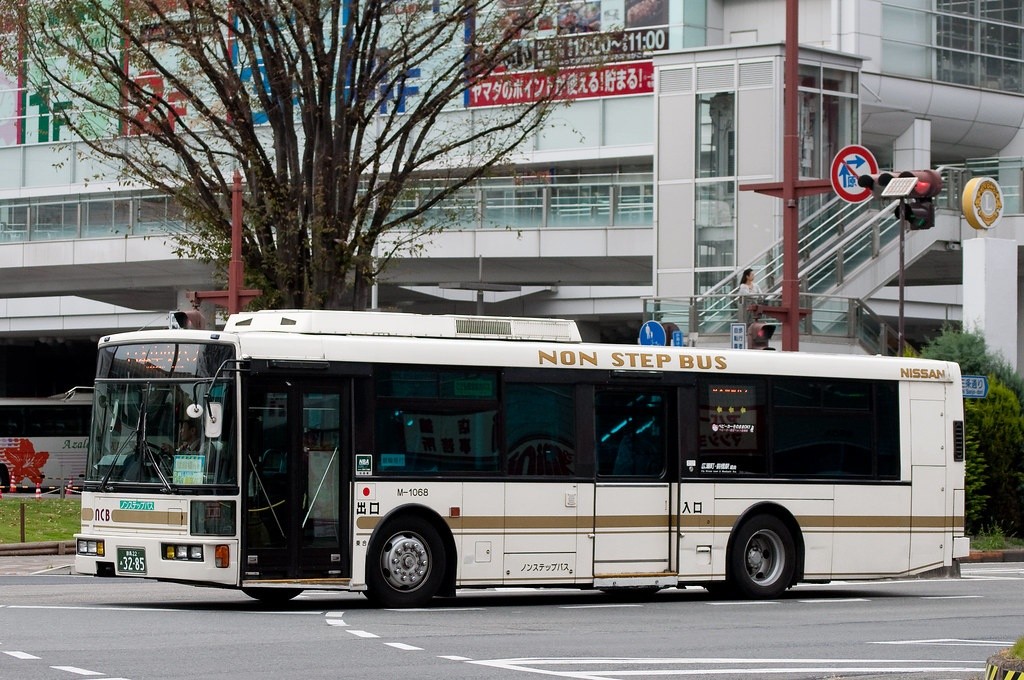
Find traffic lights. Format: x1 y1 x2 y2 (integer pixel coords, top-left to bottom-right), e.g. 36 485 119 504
174 310 206 329
858 170 942 232
747 322 777 351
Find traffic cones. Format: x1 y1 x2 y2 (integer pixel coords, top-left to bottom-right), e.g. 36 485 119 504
35 482 42 498
10 472 18 493
65 475 73 495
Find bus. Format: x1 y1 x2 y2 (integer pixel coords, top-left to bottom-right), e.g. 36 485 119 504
0 295 187 491
71 308 971 605
